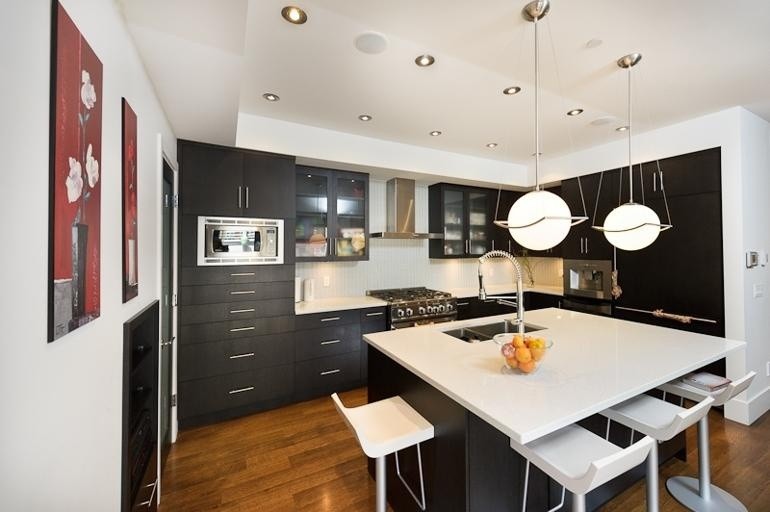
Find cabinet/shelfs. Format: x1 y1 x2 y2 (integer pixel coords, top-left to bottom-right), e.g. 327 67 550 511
176 138 385 430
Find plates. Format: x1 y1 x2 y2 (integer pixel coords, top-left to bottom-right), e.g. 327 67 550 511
470 212 486 225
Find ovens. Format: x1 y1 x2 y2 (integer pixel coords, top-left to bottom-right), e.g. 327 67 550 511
390 310 458 329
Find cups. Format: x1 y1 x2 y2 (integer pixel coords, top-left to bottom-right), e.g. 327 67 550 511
304 278 316 302
295 277 301 303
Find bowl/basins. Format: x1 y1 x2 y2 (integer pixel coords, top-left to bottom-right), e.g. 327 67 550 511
493 333 554 376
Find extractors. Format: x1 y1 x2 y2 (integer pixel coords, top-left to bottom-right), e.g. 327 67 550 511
369 178 444 239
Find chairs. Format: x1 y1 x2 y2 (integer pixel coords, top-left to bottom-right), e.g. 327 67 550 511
658 370 760 511
600 394 716 511
510 423 656 511
331 391 435 511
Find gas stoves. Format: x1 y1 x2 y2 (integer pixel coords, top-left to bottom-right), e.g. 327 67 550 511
366 286 457 319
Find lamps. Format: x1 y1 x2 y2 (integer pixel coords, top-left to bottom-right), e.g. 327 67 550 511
493 1 589 252
591 51 673 251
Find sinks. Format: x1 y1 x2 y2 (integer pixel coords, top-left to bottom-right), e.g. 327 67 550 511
468 319 539 338
442 326 490 345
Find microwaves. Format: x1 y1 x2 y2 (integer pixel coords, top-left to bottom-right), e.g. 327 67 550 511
563 258 612 300
196 216 285 267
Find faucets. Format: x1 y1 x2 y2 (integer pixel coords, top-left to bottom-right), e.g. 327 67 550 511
476 251 525 335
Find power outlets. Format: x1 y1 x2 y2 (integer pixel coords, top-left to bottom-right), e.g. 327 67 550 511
747 250 760 268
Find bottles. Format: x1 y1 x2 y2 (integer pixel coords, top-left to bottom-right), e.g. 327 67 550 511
70 187 89 321
127 191 137 286
